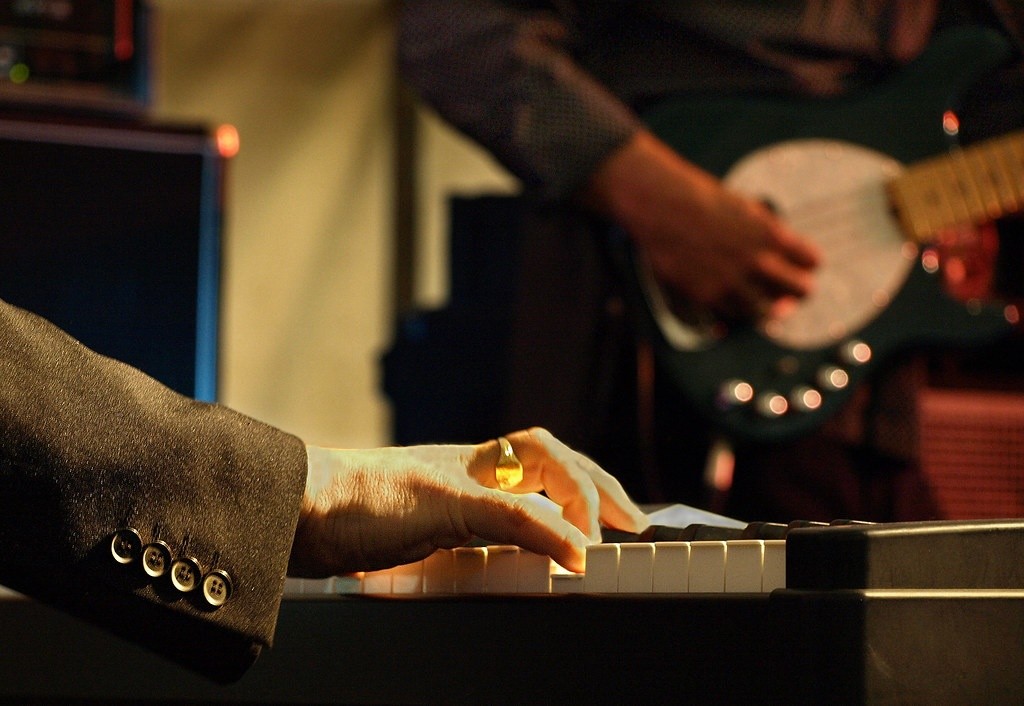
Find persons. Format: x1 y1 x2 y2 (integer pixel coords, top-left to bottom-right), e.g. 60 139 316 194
407 0 1024 518
0 303 649 684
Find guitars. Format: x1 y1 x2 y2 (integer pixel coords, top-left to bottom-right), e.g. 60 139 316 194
628 96 1024 437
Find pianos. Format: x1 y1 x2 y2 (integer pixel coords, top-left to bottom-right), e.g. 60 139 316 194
0 518 1024 706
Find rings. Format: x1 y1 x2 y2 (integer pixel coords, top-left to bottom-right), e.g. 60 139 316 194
493 435 522 488
